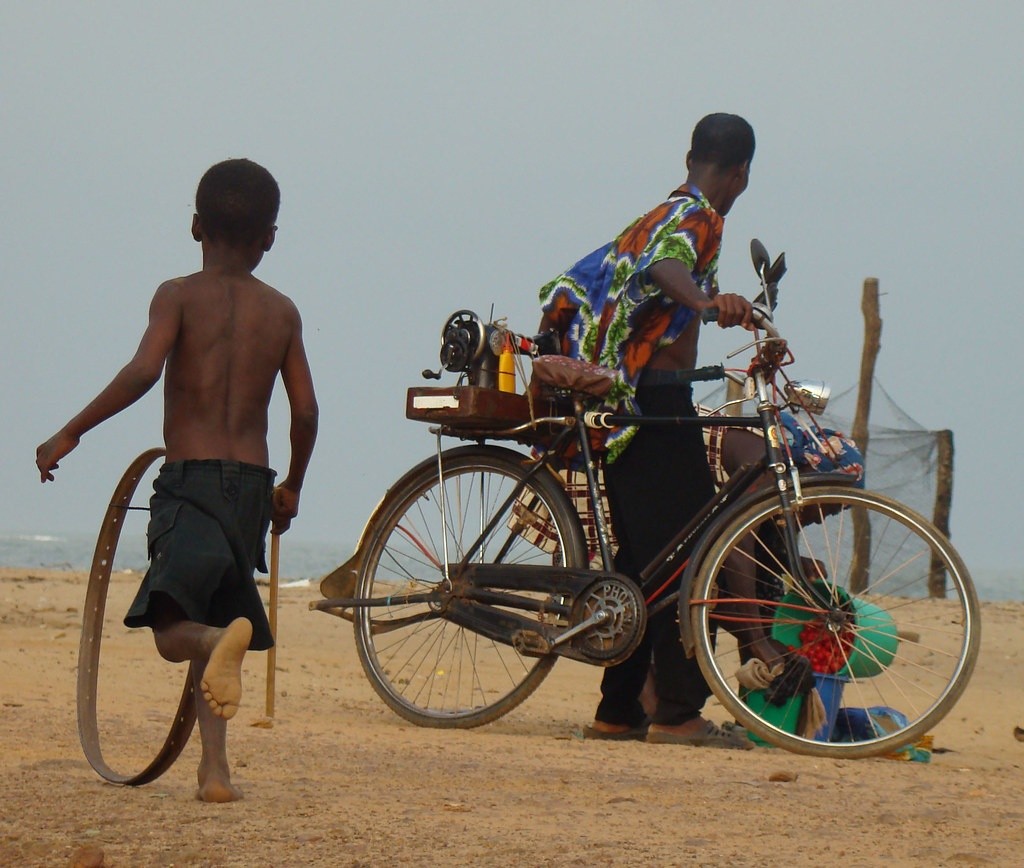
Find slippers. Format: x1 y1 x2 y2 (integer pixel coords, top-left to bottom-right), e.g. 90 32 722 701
647 720 754 751
582 720 648 741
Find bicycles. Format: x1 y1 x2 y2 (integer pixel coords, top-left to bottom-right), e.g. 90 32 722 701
306 300 984 760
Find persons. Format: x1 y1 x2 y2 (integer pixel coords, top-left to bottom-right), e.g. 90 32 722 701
34 157 318 803
507 401 866 720
538 112 758 752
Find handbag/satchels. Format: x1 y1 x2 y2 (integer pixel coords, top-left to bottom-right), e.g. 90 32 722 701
775 412 867 532
